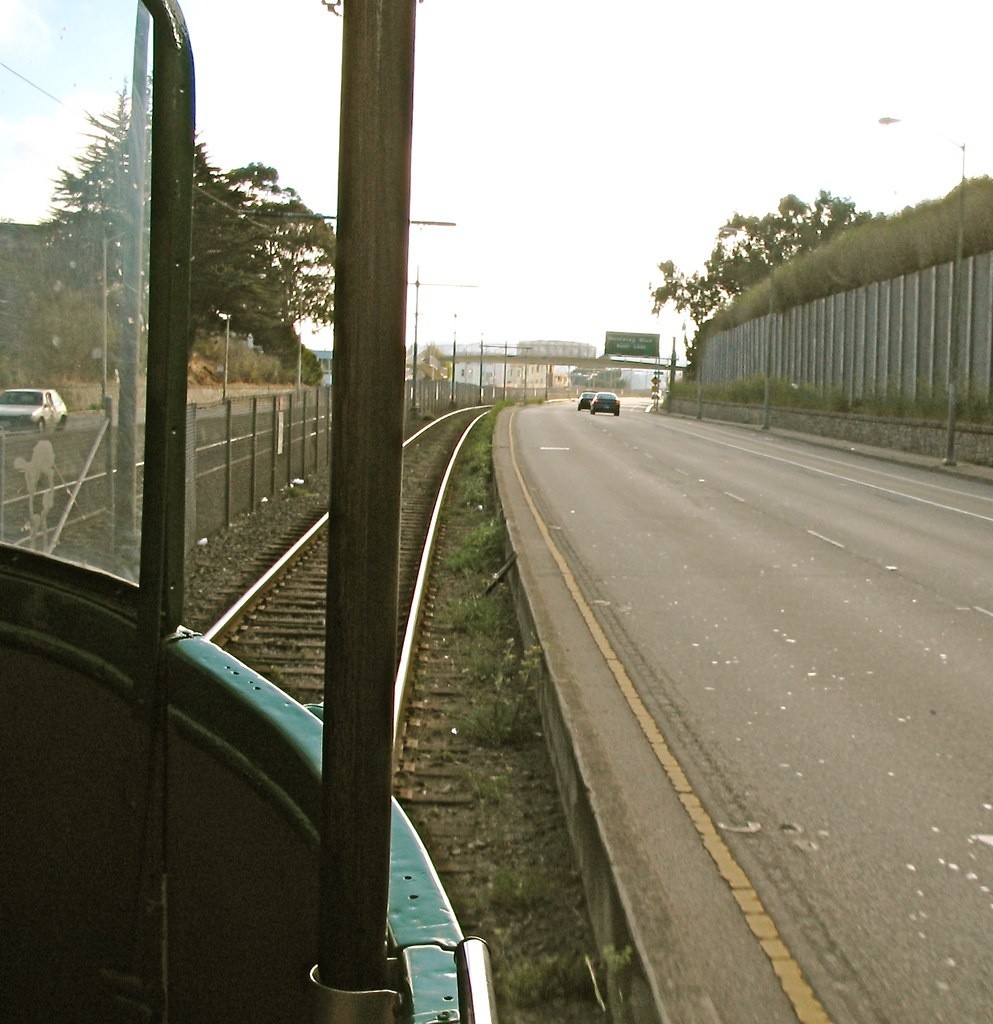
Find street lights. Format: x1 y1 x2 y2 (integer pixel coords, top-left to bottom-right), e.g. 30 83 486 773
100 225 153 409
879 115 967 466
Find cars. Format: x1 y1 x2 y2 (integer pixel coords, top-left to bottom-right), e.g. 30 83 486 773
577 392 597 412
590 392 621 416
0 388 69 434
